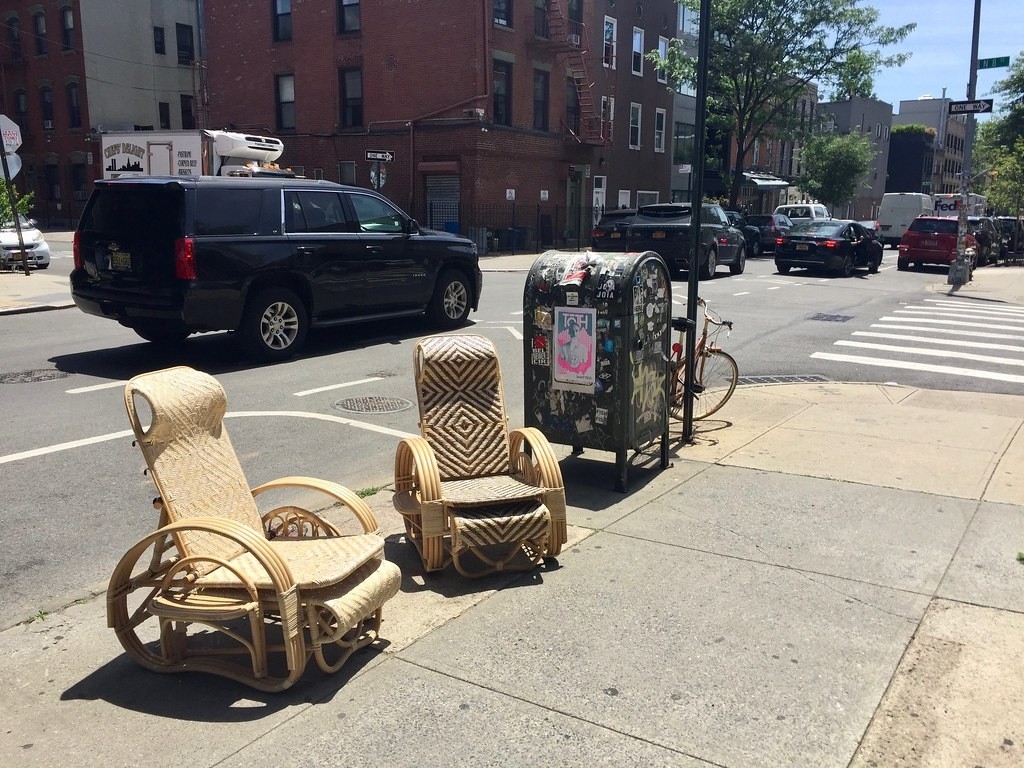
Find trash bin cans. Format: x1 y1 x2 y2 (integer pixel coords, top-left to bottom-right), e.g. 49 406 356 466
444 221 460 234
525 251 672 493
468 226 487 256
497 228 520 251
514 224 534 250
541 214 553 246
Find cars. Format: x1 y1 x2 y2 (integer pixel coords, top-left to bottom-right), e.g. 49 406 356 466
949 215 1024 267
0 212 51 270
592 208 639 252
850 220 879 241
775 219 884 278
723 210 780 258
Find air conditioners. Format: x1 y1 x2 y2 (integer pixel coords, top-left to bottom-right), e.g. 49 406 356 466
42 120 53 130
568 34 580 46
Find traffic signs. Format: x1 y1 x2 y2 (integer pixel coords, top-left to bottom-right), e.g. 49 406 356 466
948 99 993 114
363 149 397 164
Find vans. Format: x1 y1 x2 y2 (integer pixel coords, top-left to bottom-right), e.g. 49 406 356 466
773 204 833 225
101 126 309 180
876 192 935 250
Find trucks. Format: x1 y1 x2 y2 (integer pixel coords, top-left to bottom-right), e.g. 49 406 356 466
932 193 994 218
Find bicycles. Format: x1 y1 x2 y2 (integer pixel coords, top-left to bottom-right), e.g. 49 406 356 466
669 293 739 422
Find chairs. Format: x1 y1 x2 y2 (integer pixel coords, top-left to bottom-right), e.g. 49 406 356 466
391 335 568 573
106 366 402 691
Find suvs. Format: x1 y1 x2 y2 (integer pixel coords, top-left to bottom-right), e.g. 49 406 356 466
897 213 980 272
626 203 748 281
69 176 483 362
